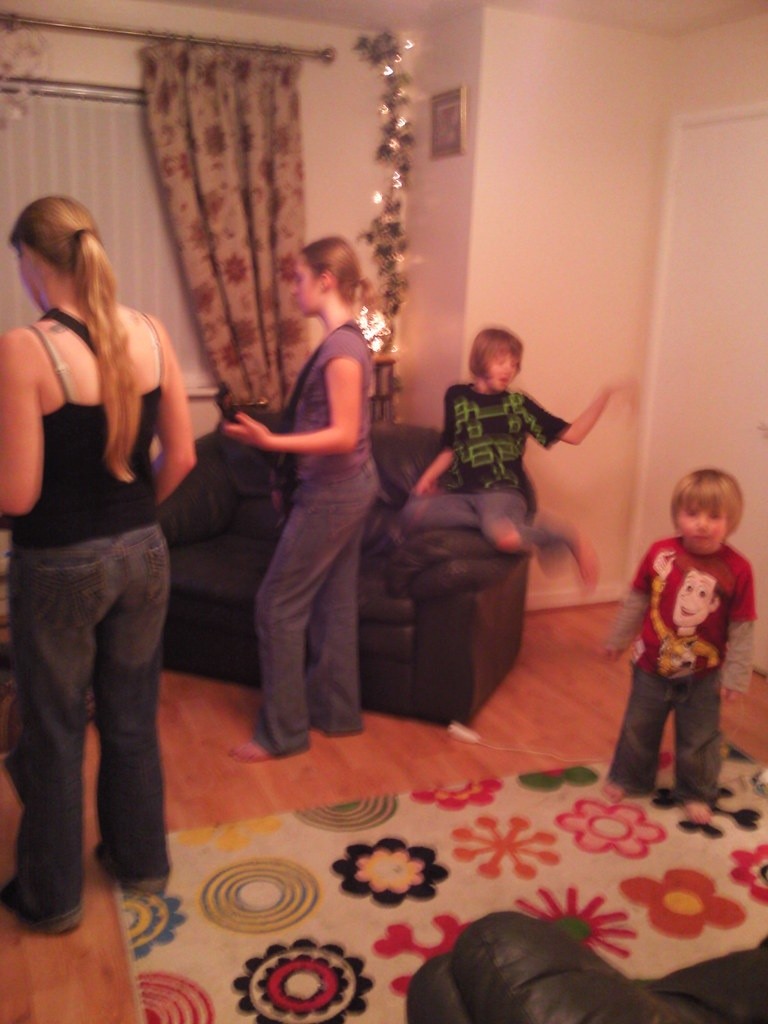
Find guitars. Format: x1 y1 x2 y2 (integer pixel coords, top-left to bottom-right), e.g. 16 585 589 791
214 381 305 525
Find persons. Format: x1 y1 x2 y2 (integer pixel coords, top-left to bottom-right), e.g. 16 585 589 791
398 328 625 589
0 196 198 935
221 236 381 764
598 469 758 824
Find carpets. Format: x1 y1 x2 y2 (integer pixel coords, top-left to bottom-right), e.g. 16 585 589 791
110 732 767 1023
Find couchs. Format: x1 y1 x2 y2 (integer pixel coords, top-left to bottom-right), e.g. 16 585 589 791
159 421 535 729
406 911 768 1024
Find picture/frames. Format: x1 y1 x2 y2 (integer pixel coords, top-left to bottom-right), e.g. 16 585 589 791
426 83 468 158
